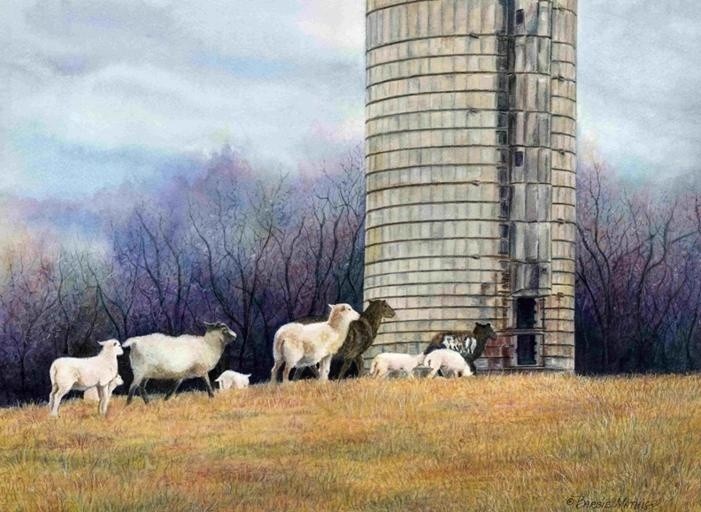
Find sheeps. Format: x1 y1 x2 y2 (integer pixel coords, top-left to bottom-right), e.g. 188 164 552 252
49 339 124 416
125 320 238 407
266 299 498 385
217 369 253 390
81 373 124 403
